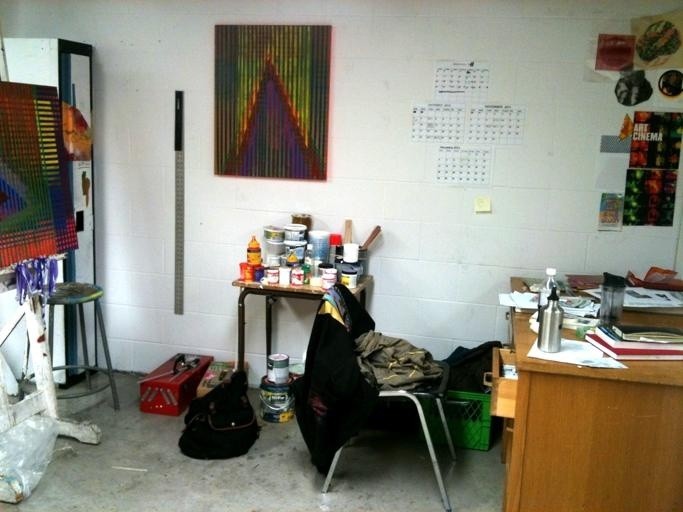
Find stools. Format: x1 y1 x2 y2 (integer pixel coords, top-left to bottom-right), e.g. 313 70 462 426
42 278 123 414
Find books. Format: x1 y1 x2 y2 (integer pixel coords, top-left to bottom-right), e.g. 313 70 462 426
515 308 683 361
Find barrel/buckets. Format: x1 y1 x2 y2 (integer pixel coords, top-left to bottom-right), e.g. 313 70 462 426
259 377 295 423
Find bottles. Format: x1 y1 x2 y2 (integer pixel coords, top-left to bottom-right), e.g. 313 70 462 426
537 286 562 353
278 234 363 290
601 270 624 330
538 268 568 305
248 236 259 248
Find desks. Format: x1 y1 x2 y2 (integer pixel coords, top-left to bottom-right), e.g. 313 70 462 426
482 274 679 510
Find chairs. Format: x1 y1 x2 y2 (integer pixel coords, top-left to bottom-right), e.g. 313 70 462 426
320 277 457 511
231 248 374 371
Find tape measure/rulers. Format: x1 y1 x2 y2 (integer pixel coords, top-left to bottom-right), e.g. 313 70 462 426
174 90 184 315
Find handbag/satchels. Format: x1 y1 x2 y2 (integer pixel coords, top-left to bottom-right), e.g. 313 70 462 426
178 369 264 461
